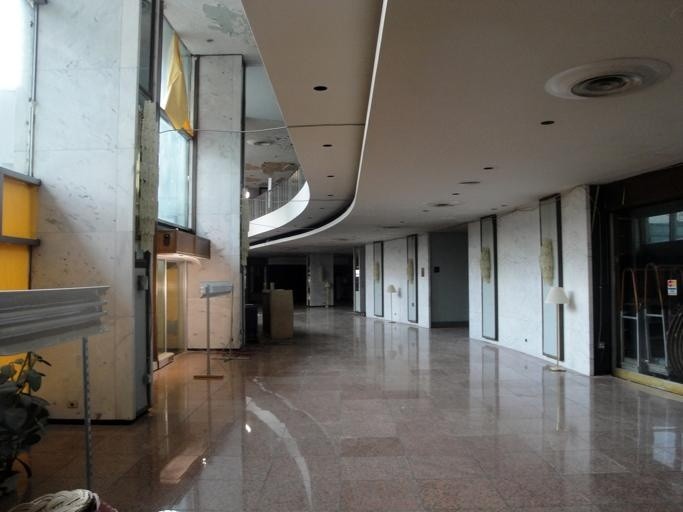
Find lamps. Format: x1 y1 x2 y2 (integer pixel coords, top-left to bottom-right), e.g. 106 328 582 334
543 287 570 372
386 285 397 323
